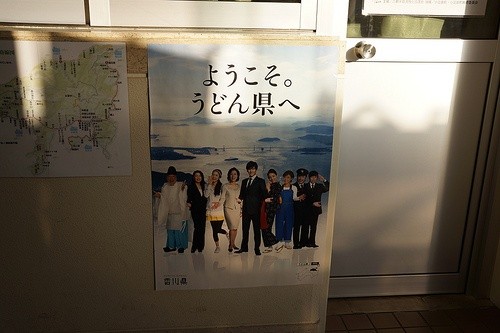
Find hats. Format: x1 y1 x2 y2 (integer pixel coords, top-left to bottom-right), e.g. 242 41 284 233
297 169 308 177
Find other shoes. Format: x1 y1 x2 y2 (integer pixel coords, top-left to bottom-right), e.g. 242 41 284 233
214 246 220 253
163 247 176 252
178 248 184 253
262 246 273 253
255 247 261 255
234 249 248 253
285 243 292 249
294 242 319 249
190 248 202 253
276 244 284 253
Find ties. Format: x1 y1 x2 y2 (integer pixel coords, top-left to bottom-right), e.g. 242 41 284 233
247 178 252 187
300 184 303 188
312 185 314 188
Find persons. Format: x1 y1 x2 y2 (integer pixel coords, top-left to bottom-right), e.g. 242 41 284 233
292 168 310 249
275 170 306 253
153 166 188 255
305 170 329 247
211 167 242 252
187 170 208 255
204 169 229 253
261 169 282 253
235 161 273 256
